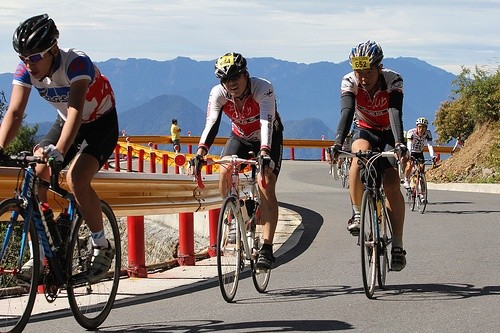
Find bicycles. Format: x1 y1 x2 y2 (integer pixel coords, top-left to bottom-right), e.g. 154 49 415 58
0 147 122 333
406 156 439 214
327 147 349 188
193 154 274 303
325 145 409 299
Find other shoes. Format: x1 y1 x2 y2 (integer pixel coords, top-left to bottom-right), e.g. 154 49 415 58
227 223 237 243
403 181 412 189
390 247 407 272
419 196 427 202
347 213 361 234
257 249 276 271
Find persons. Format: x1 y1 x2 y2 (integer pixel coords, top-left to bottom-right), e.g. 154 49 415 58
324 41 443 271
187 52 285 269
0 14 119 283
170 119 182 153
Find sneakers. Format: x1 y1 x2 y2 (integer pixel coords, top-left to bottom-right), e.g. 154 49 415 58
14 258 48 284
86 239 115 283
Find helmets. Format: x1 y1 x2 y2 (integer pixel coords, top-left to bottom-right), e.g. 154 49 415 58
415 117 429 127
214 52 248 79
12 14 59 55
349 39 384 71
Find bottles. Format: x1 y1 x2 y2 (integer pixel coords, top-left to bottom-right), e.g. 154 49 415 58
376 199 380 216
40 202 63 248
239 200 249 225
245 196 255 220
57 206 71 255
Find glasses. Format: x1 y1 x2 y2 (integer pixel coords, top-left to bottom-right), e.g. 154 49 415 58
18 43 58 62
